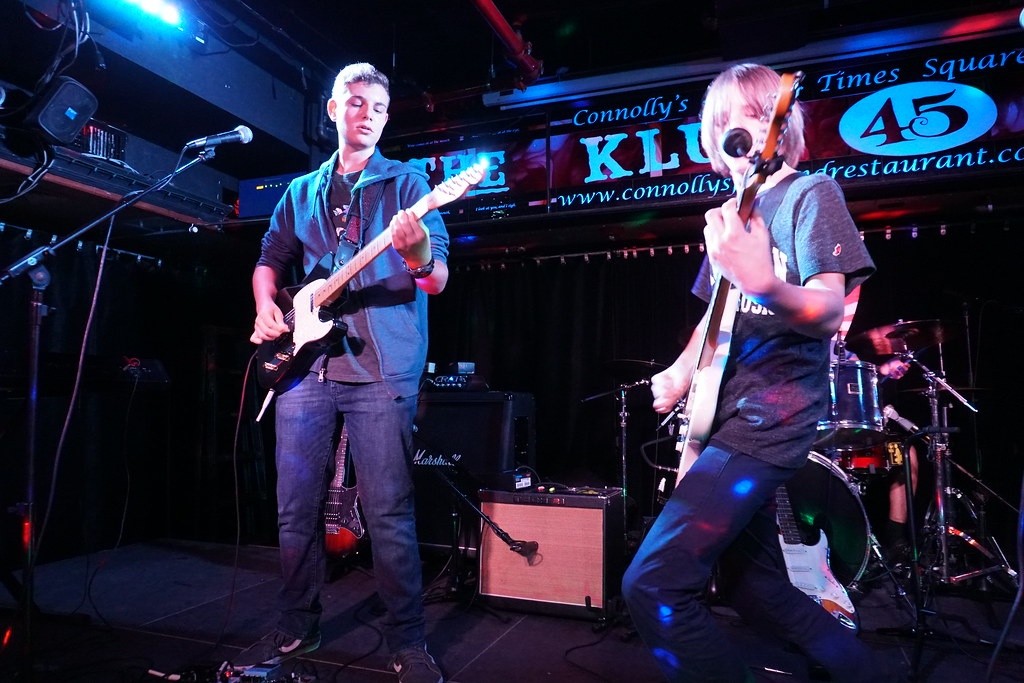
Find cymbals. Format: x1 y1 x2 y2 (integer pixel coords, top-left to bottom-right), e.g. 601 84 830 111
896 383 989 396
607 354 667 381
845 317 975 358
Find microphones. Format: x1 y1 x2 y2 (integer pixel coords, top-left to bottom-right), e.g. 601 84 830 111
186 125 253 148
509 540 539 556
722 128 755 165
884 403 930 440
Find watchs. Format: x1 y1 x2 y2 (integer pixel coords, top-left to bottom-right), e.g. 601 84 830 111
402 256 435 279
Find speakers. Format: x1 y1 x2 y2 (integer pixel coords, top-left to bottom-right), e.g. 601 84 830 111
475 487 626 619
409 392 539 556
26 75 99 145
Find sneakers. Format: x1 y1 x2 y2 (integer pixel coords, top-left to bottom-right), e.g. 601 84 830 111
386 638 443 683
227 625 321 669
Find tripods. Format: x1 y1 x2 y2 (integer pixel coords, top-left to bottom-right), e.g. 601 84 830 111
875 321 1012 682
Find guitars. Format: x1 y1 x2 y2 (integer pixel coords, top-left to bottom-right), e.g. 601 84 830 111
671 66 803 486
255 160 486 392
771 476 859 633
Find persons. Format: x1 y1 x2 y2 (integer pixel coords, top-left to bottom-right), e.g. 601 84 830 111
622 63 908 683
851 352 919 541
234 62 450 683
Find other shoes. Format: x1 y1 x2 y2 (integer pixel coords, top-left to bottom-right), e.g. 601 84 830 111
885 539 914 564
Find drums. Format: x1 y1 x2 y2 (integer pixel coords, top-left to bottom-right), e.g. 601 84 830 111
805 449 872 591
815 359 886 436
